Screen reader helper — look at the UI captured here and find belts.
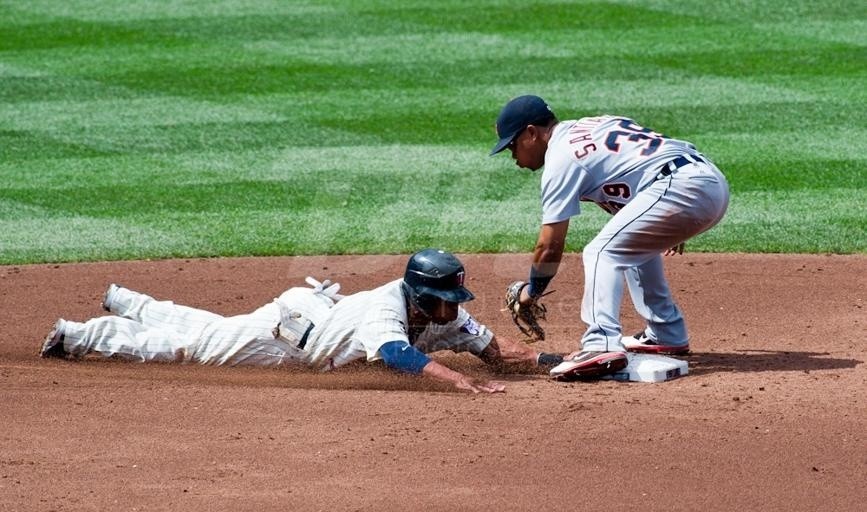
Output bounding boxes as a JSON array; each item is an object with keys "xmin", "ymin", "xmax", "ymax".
[
  {"xmin": 655, "ymin": 154, "xmax": 709, "ymax": 181},
  {"xmin": 295, "ymin": 322, "xmax": 314, "ymax": 351}
]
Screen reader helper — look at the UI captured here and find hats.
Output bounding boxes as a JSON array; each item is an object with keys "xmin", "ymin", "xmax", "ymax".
[{"xmin": 486, "ymin": 96, "xmax": 556, "ymax": 156}]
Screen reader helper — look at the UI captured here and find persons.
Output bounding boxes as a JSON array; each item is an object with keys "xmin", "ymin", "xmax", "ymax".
[
  {"xmin": 488, "ymin": 91, "xmax": 732, "ymax": 381},
  {"xmin": 40, "ymin": 244, "xmax": 571, "ymax": 394}
]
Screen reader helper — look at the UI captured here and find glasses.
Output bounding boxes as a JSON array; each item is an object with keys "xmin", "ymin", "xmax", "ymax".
[{"xmin": 507, "ymin": 130, "xmax": 524, "ymax": 151}]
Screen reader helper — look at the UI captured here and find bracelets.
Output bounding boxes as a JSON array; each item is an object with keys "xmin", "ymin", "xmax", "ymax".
[
  {"xmin": 526, "ymin": 266, "xmax": 557, "ymax": 298},
  {"xmin": 536, "ymin": 350, "xmax": 571, "ymax": 369}
]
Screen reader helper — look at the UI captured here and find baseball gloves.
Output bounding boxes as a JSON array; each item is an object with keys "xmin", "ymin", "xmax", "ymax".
[{"xmin": 505, "ymin": 281, "xmax": 556, "ymax": 344}]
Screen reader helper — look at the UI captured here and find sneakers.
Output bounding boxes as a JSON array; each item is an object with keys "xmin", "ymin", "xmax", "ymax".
[
  {"xmin": 549, "ymin": 347, "xmax": 628, "ymax": 383},
  {"xmin": 39, "ymin": 317, "xmax": 69, "ymax": 358},
  {"xmin": 100, "ymin": 282, "xmax": 121, "ymax": 312},
  {"xmin": 618, "ymin": 330, "xmax": 691, "ymax": 356}
]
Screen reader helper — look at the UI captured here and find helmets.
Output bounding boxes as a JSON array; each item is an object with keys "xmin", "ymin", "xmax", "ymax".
[{"xmin": 402, "ymin": 248, "xmax": 474, "ymax": 305}]
